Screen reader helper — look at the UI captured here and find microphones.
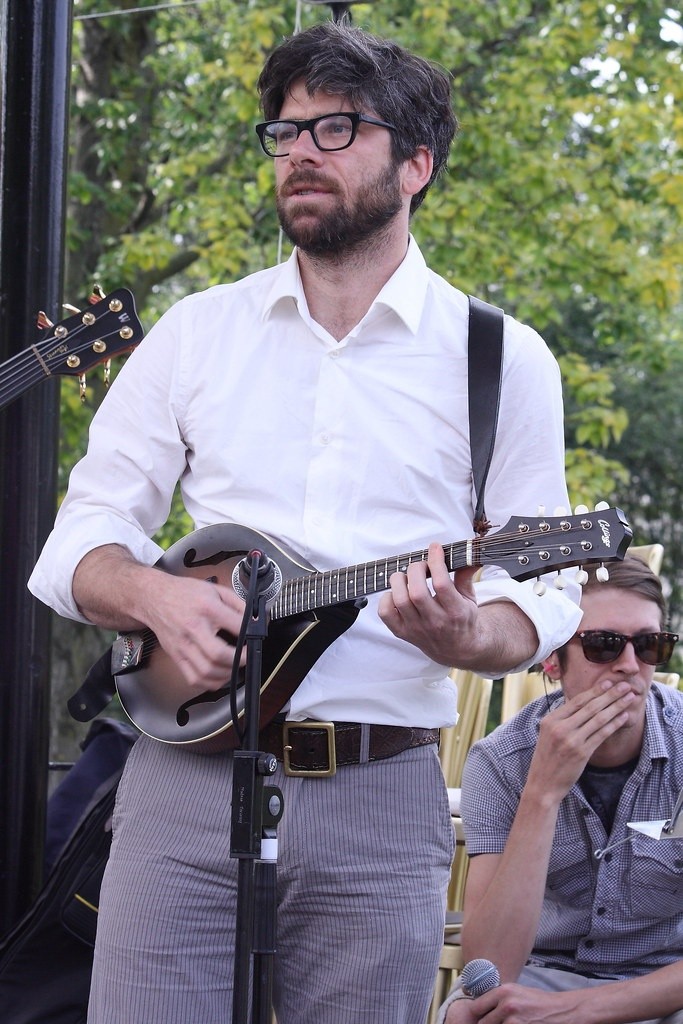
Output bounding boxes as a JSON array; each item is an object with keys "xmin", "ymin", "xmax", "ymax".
[
  {"xmin": 461, "ymin": 958, "xmax": 501, "ymax": 996},
  {"xmin": 232, "ymin": 548, "xmax": 283, "ymax": 604}
]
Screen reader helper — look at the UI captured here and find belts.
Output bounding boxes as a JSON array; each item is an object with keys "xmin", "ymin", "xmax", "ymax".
[{"xmin": 261, "ymin": 725, "xmax": 441, "ymax": 776}]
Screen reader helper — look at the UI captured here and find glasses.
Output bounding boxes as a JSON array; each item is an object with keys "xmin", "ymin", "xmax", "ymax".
[
  {"xmin": 255, "ymin": 110, "xmax": 395, "ymax": 158},
  {"xmin": 575, "ymin": 629, "xmax": 679, "ymax": 666}
]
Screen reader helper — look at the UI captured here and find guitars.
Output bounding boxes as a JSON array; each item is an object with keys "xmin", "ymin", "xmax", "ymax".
[
  {"xmin": 0, "ymin": 283, "xmax": 145, "ymax": 415},
  {"xmin": 114, "ymin": 498, "xmax": 635, "ymax": 758}
]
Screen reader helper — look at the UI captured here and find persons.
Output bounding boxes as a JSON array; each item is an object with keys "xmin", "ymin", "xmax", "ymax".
[
  {"xmin": 26, "ymin": 19, "xmax": 586, "ymax": 1024},
  {"xmin": 434, "ymin": 555, "xmax": 683, "ymax": 1024}
]
